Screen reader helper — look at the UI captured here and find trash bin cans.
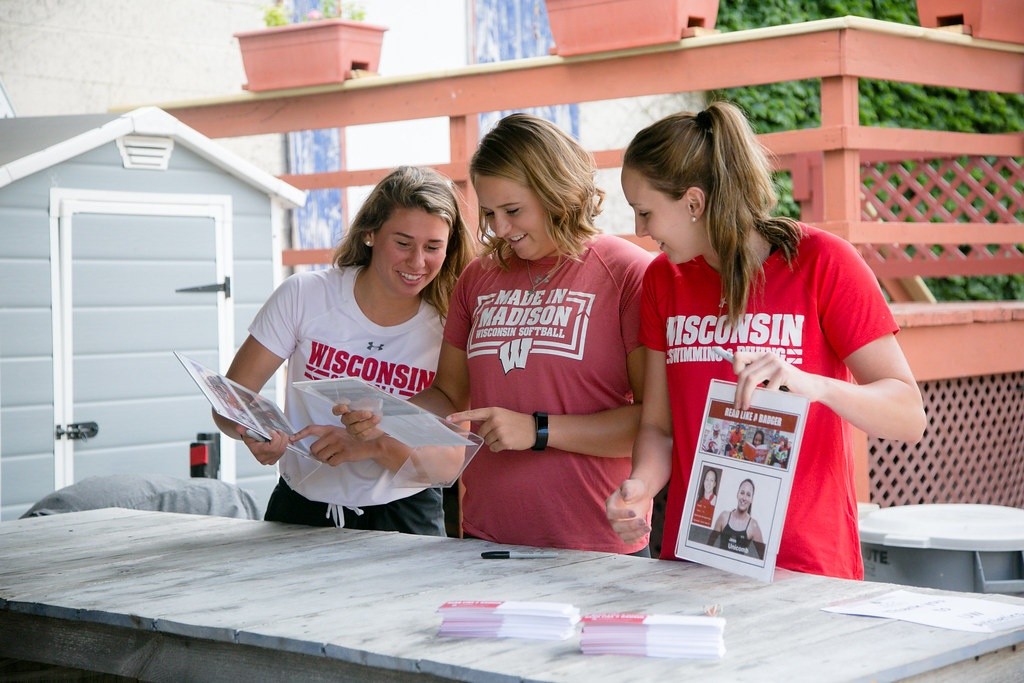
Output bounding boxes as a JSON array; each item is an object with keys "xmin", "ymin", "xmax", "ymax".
[{"xmin": 857, "ymin": 502, "xmax": 1024, "ymax": 598}]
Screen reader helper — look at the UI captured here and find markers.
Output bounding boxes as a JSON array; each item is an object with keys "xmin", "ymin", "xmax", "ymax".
[
  {"xmin": 482, "ymin": 549, "xmax": 560, "ymax": 559},
  {"xmin": 712, "ymin": 345, "xmax": 789, "ymax": 391}
]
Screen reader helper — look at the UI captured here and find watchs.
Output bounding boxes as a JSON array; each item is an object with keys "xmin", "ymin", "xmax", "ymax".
[{"xmin": 533, "ymin": 411, "xmax": 549, "ymax": 452}]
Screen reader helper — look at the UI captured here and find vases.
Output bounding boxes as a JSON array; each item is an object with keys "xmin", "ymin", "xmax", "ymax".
[
  {"xmin": 232, "ymin": 17, "xmax": 388, "ymax": 93},
  {"xmin": 545, "ymin": 0, "xmax": 720, "ymax": 55},
  {"xmin": 913, "ymin": 0, "xmax": 1024, "ymax": 45}
]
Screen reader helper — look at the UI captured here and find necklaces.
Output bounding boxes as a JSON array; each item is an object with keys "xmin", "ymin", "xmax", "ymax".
[{"xmin": 527, "ymin": 238, "xmax": 583, "ymax": 296}]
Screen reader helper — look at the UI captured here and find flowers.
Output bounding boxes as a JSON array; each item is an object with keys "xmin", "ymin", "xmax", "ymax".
[{"xmin": 261, "ymin": 2, "xmax": 366, "ymax": 26}]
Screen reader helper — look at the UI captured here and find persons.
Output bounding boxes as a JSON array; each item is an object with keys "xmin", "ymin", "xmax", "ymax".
[
  {"xmin": 703, "ymin": 422, "xmax": 722, "ymax": 455},
  {"xmin": 211, "ymin": 166, "xmax": 475, "ymax": 537},
  {"xmin": 692, "ymin": 468, "xmax": 717, "ymax": 527},
  {"xmin": 604, "ymin": 101, "xmax": 926, "ymax": 580},
  {"xmin": 770, "ymin": 438, "xmax": 791, "ymax": 469},
  {"xmin": 708, "ymin": 479, "xmax": 766, "ymax": 560},
  {"xmin": 724, "ymin": 424, "xmax": 742, "ymax": 456},
  {"xmin": 741, "ymin": 431, "xmax": 764, "ymax": 460},
  {"xmin": 333, "ymin": 112, "xmax": 656, "ymax": 560}
]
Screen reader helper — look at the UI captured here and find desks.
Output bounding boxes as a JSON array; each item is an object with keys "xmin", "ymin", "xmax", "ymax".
[{"xmin": 0, "ymin": 507, "xmax": 1024, "ymax": 683}]
